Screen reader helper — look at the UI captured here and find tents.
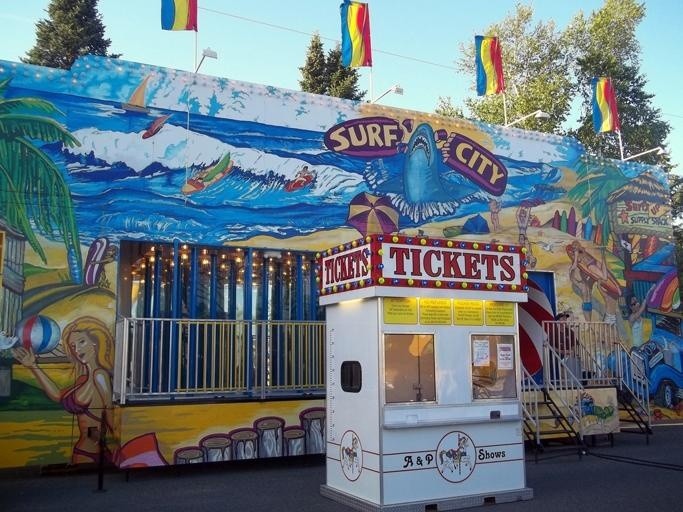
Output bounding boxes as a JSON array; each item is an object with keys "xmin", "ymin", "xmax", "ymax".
[{"xmin": 459, "ymin": 214, "xmax": 489, "ymax": 234}]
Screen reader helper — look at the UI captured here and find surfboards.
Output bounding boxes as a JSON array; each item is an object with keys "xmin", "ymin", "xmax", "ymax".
[
  {"xmin": 142, "ymin": 116, "xmax": 168, "ymax": 138},
  {"xmin": 566, "ymin": 244, "xmax": 622, "ymax": 297},
  {"xmin": 285, "ymin": 175, "xmax": 313, "ymax": 192},
  {"xmin": 182, "ymin": 160, "xmax": 234, "ymax": 192}
]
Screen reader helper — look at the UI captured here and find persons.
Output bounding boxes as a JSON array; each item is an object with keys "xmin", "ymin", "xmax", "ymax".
[
  {"xmin": 290, "ymin": 165, "xmax": 309, "ymax": 188},
  {"xmin": 488, "ymin": 199, "xmax": 501, "ymax": 233},
  {"xmin": 514, "ymin": 203, "xmax": 529, "ymax": 237},
  {"xmin": 6, "ymin": 315, "xmax": 116, "ymax": 465},
  {"xmin": 594, "ymin": 279, "xmax": 620, "ymax": 334},
  {"xmin": 415, "ymin": 229, "xmax": 429, "ymax": 238},
  {"xmin": 625, "ymin": 282, "xmax": 658, "ymax": 351},
  {"xmin": 568, "ymin": 239, "xmax": 624, "ymax": 298},
  {"xmin": 88, "ymin": 244, "xmax": 118, "ymax": 288},
  {"xmin": 522, "ymin": 239, "xmax": 538, "ymax": 268},
  {"xmin": 517, "ymin": 233, "xmax": 527, "ymax": 254},
  {"xmin": 568, "ymin": 267, "xmax": 600, "ymax": 322}
]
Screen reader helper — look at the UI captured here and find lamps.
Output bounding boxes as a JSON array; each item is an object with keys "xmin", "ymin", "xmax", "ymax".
[{"xmin": 194, "ymin": 47, "xmax": 217, "ymax": 74}]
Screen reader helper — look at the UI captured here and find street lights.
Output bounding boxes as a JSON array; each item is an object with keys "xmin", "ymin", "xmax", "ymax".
[{"xmin": 195, "ymin": 48, "xmax": 218, "ymax": 71}]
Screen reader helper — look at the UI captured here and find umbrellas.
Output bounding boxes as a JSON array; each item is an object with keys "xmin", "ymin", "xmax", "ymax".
[{"xmin": 344, "ymin": 191, "xmax": 401, "ymax": 238}]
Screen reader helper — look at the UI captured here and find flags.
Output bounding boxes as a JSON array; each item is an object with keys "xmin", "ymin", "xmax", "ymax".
[
  {"xmin": 158, "ymin": 0, "xmax": 200, "ymax": 33},
  {"xmin": 473, "ymin": 35, "xmax": 504, "ymax": 97},
  {"xmin": 335, "ymin": 0, "xmax": 373, "ymax": 69},
  {"xmin": 586, "ymin": 75, "xmax": 621, "ymax": 133}
]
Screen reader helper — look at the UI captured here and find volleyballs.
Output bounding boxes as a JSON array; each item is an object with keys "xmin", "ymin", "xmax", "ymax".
[{"xmin": 18, "ymin": 314, "xmax": 60, "ymax": 355}]
